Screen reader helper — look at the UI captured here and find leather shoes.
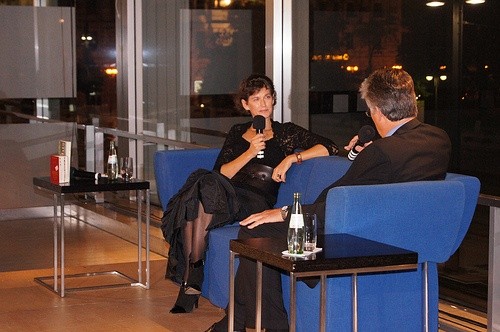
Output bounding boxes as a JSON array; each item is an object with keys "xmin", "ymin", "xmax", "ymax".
[{"xmin": 205, "ymin": 322, "xmax": 246, "ymax": 332}]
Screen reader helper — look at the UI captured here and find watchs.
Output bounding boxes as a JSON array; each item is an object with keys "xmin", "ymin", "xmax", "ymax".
[{"xmin": 281, "ymin": 205, "xmax": 289, "ymax": 220}]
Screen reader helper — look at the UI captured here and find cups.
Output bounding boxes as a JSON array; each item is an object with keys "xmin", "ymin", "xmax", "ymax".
[
  {"xmin": 120, "ymin": 157, "xmax": 133, "ymax": 182},
  {"xmin": 303, "ymin": 212, "xmax": 317, "ymax": 251}
]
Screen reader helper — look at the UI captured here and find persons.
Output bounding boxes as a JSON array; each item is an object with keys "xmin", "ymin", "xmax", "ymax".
[
  {"xmin": 161, "ymin": 74, "xmax": 338, "ymax": 313},
  {"xmin": 205, "ymin": 67, "xmax": 451, "ymax": 332}
]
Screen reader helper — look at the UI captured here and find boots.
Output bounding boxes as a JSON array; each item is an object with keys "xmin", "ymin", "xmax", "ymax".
[
  {"xmin": 183, "ymin": 253, "xmax": 206, "ymax": 295},
  {"xmin": 170, "ymin": 280, "xmax": 200, "ymax": 314}
]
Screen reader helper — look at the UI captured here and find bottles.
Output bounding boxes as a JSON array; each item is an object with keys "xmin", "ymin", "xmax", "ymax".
[
  {"xmin": 107, "ymin": 140, "xmax": 118, "ymax": 180},
  {"xmin": 287, "ymin": 192, "xmax": 305, "ymax": 255}
]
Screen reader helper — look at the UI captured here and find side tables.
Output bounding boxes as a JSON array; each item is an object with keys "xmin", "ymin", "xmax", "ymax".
[
  {"xmin": 32, "ymin": 174, "xmax": 151, "ymax": 298},
  {"xmin": 228, "ymin": 233, "xmax": 419, "ymax": 332}
]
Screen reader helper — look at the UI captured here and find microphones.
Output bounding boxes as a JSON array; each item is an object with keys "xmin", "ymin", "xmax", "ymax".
[
  {"xmin": 253, "ymin": 115, "xmax": 266, "ymax": 159},
  {"xmin": 69, "ymin": 167, "xmax": 101, "ymax": 179},
  {"xmin": 348, "ymin": 125, "xmax": 375, "ymax": 161}
]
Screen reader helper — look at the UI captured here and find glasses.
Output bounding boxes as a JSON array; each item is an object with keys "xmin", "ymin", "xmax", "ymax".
[{"xmin": 365, "ymin": 106, "xmax": 372, "ymax": 117}]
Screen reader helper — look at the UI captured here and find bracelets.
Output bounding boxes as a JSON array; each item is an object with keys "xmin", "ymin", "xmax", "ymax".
[{"xmin": 296, "ymin": 152, "xmax": 301, "ymax": 162}]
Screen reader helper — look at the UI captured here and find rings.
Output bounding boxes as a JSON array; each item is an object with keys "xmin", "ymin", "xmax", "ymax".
[{"xmin": 277, "ymin": 174, "xmax": 281, "ymax": 179}]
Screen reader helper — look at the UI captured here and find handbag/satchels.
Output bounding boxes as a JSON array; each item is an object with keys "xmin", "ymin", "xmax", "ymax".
[{"xmin": 237, "ymin": 163, "xmax": 273, "ymax": 189}]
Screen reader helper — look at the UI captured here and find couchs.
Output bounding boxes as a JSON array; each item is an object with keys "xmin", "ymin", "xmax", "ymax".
[{"xmin": 152, "ymin": 148, "xmax": 481, "ymax": 332}]
